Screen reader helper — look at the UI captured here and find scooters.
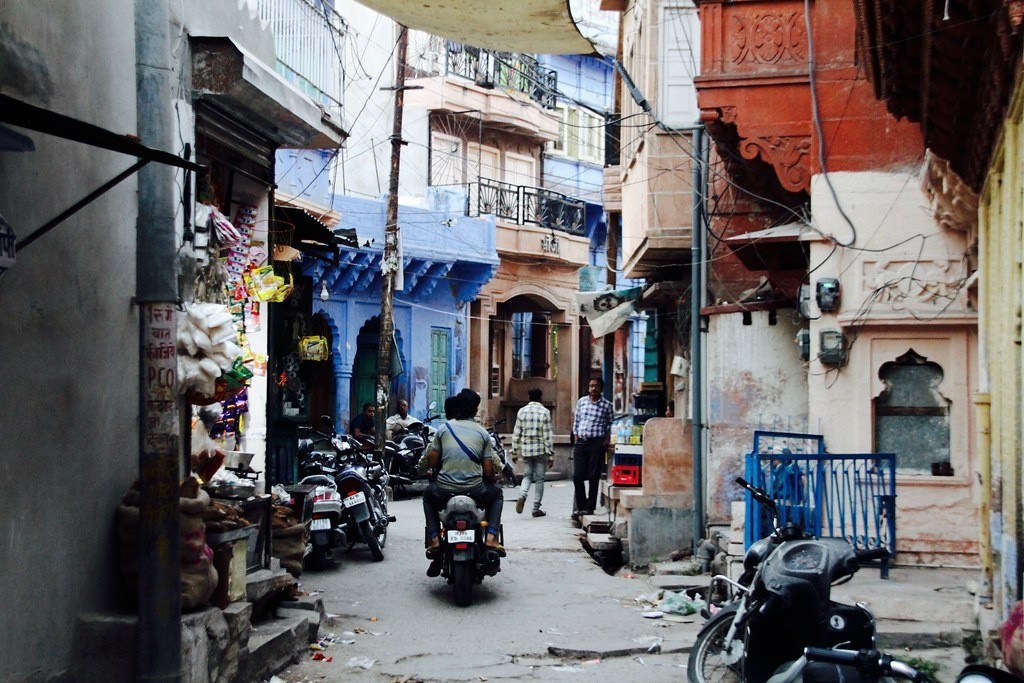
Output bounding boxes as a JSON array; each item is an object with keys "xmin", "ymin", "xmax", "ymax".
[
  {"xmin": 685, "ymin": 476, "xmax": 1024, "ymax": 683},
  {"xmin": 485, "ymin": 417, "xmax": 520, "ymax": 487}
]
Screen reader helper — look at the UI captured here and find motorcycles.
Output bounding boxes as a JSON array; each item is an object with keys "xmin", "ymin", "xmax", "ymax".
[
  {"xmin": 296, "ymin": 414, "xmax": 397, "ymax": 568},
  {"xmin": 427, "ymin": 489, "xmax": 506, "ymax": 607},
  {"xmin": 357, "ymin": 401, "xmax": 442, "ymax": 497}
]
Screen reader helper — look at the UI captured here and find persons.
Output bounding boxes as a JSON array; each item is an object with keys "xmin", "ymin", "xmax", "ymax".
[
  {"xmin": 385, "ymin": 399, "xmax": 437, "ymax": 440},
  {"xmin": 350, "ymin": 403, "xmax": 375, "ymax": 443},
  {"xmin": 1000, "ymin": 599, "xmax": 1024, "ymax": 677},
  {"xmin": 512, "ymin": 388, "xmax": 554, "ymax": 517},
  {"xmin": 771, "ymin": 445, "xmax": 806, "ymax": 501},
  {"xmin": 573, "ymin": 376, "xmax": 613, "ymax": 513},
  {"xmin": 416, "ymin": 389, "xmax": 506, "ymax": 577},
  {"xmin": 665, "ymin": 400, "xmax": 674, "ymax": 418}
]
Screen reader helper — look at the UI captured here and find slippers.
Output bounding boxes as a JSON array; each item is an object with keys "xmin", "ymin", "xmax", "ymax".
[
  {"xmin": 426, "ymin": 544, "xmax": 440, "ymax": 559},
  {"xmin": 490, "ymin": 543, "xmax": 506, "ymax": 557}
]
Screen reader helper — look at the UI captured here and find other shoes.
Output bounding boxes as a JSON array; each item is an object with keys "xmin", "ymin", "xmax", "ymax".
[
  {"xmin": 516, "ymin": 496, "xmax": 525, "ymax": 514},
  {"xmin": 533, "ymin": 510, "xmax": 545, "ymax": 517},
  {"xmin": 427, "ymin": 559, "xmax": 443, "ymax": 577}
]
